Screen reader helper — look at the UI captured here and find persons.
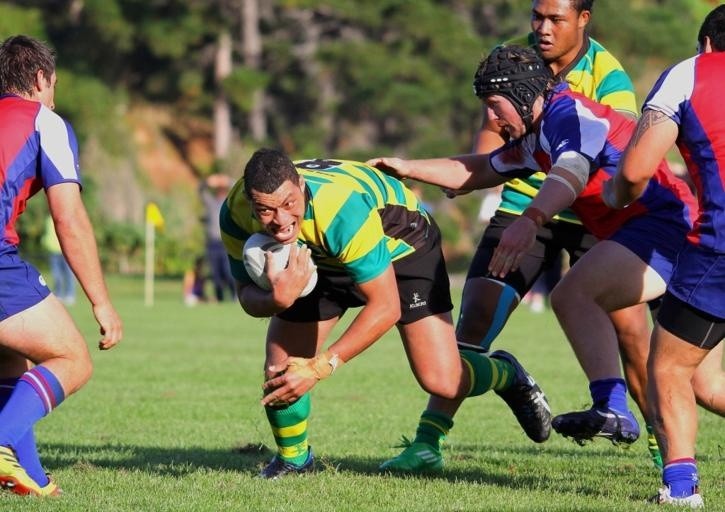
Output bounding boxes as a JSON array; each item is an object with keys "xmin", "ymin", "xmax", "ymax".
[
  {"xmin": 375, "ymin": 0, "xmax": 673, "ymax": 476},
  {"xmin": 219, "ymin": 147, "xmax": 556, "ymax": 483},
  {"xmin": 365, "ymin": 40, "xmax": 724, "ymax": 446},
  {"xmin": 201, "ymin": 171, "xmax": 234, "ymax": 307},
  {"xmin": 602, "ymin": 0, "xmax": 725, "ymax": 511},
  {"xmin": 182, "ymin": 256, "xmax": 209, "ymax": 305},
  {"xmin": 1, "ymin": 34, "xmax": 123, "ymax": 498}
]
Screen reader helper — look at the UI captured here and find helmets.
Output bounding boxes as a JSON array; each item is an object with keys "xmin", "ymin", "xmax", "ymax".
[{"xmin": 472, "ymin": 43, "xmax": 552, "ymax": 122}]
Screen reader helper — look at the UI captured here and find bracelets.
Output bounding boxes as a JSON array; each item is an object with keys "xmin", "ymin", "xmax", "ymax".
[
  {"xmin": 521, "ymin": 206, "xmax": 548, "ymax": 229},
  {"xmin": 610, "ymin": 193, "xmax": 629, "ymax": 209}
]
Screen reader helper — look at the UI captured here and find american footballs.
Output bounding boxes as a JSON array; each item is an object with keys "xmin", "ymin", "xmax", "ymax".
[{"xmin": 242, "ymin": 231, "xmax": 319, "ymax": 299}]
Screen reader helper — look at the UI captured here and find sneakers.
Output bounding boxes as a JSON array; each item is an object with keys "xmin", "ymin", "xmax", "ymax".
[
  {"xmin": 256, "ymin": 448, "xmax": 316, "ymax": 481},
  {"xmin": 651, "ymin": 489, "xmax": 704, "ymax": 507},
  {"xmin": 378, "ymin": 434, "xmax": 445, "ymax": 476},
  {"xmin": 490, "ymin": 348, "xmax": 551, "ymax": 442},
  {"xmin": 551, "ymin": 402, "xmax": 638, "ymax": 448},
  {"xmin": 647, "ymin": 425, "xmax": 664, "ymax": 471},
  {"xmin": 0, "ymin": 445, "xmax": 66, "ymax": 497}
]
